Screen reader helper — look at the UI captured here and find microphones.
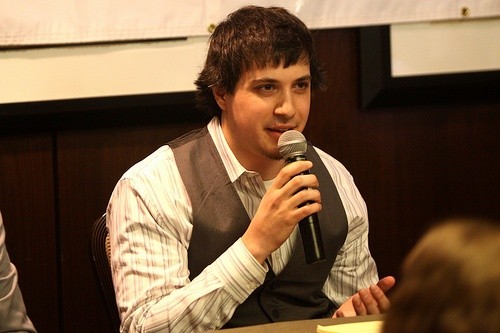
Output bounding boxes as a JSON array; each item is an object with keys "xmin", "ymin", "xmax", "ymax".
[{"xmin": 278, "ymin": 130, "xmax": 327, "ymax": 264}]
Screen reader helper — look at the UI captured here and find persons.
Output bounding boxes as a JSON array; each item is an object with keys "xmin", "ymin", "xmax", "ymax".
[{"xmin": 105, "ymin": 6, "xmax": 395, "ymax": 333}]
